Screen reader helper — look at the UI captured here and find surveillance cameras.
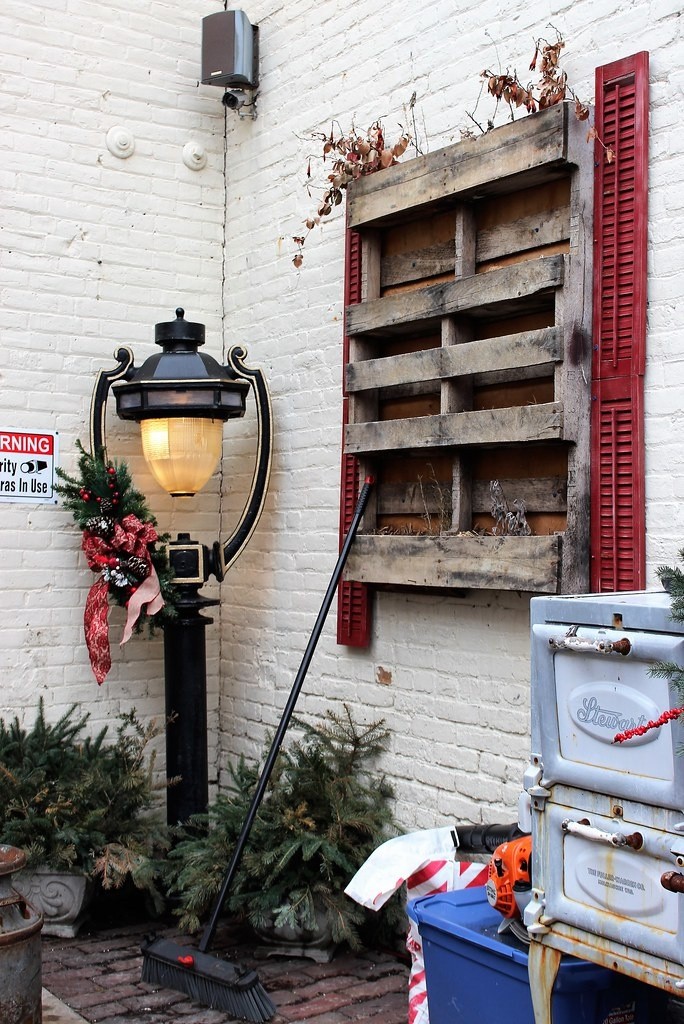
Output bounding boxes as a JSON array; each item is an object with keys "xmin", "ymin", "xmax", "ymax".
[{"xmin": 222, "ymin": 89, "xmax": 248, "ymax": 110}]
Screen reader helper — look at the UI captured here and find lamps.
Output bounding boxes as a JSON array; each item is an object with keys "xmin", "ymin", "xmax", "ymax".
[{"xmin": 111, "ymin": 307, "xmax": 250, "ymax": 497}]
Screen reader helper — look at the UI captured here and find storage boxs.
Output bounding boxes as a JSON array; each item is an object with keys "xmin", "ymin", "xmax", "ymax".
[{"xmin": 405, "ymin": 887, "xmax": 642, "ymax": 1024}]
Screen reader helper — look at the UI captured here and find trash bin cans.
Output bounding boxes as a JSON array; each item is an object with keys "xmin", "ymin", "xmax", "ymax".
[{"xmin": 406, "ymin": 885, "xmax": 637, "ymax": 1024}]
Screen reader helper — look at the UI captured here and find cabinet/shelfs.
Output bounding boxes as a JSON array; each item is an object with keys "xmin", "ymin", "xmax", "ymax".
[{"xmin": 512, "ymin": 586, "xmax": 684, "ymax": 1023}]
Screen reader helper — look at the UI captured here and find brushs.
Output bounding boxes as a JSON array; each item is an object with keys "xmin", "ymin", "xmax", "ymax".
[{"xmin": 139, "ymin": 476, "xmax": 372, "ymax": 1023}]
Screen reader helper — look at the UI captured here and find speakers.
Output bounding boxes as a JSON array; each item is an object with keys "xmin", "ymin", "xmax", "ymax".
[{"xmin": 202, "ymin": 10, "xmax": 253, "ymax": 86}]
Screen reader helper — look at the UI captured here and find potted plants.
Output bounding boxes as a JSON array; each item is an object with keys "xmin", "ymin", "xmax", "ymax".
[
  {"xmin": 0, "ymin": 693, "xmax": 182, "ymax": 940},
  {"xmin": 135, "ymin": 700, "xmax": 413, "ymax": 964}
]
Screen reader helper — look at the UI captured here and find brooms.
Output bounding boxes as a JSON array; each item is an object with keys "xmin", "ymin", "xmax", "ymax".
[{"xmin": 142, "ymin": 478, "xmax": 375, "ymax": 1022}]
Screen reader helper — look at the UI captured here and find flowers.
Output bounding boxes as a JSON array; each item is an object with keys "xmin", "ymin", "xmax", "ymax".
[{"xmin": 49, "ymin": 437, "xmax": 181, "ymax": 684}]
